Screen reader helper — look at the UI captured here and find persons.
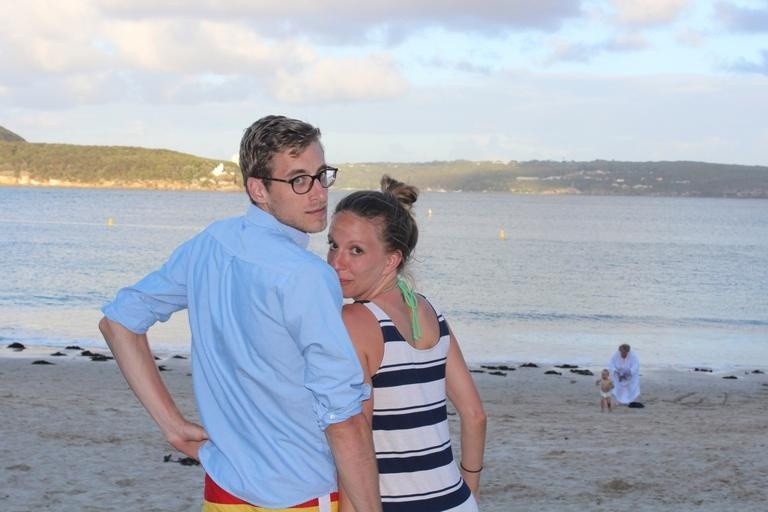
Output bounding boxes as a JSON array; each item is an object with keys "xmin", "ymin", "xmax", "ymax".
[
  {"xmin": 596, "ymin": 370, "xmax": 616, "ymax": 412},
  {"xmin": 606, "ymin": 342, "xmax": 642, "ymax": 407},
  {"xmin": 98, "ymin": 115, "xmax": 384, "ymax": 511},
  {"xmin": 326, "ymin": 171, "xmax": 489, "ymax": 512}
]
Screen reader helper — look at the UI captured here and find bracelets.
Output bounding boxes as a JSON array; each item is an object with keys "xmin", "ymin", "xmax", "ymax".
[{"xmin": 460, "ymin": 461, "xmax": 484, "ymax": 474}]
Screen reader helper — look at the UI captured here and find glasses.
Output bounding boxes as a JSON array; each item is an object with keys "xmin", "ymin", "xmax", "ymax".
[{"xmin": 245, "ymin": 165, "xmax": 339, "ymax": 198}]
